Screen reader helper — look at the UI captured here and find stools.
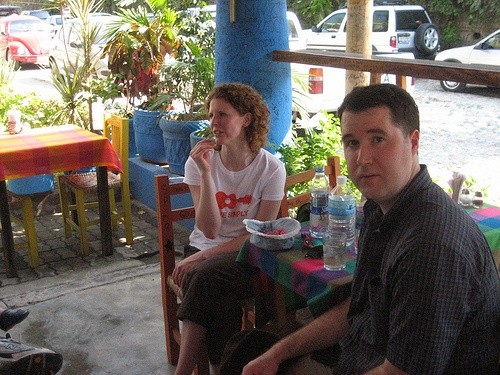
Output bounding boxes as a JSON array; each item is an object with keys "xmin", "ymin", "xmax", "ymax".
[{"xmin": 6, "ymin": 174, "xmax": 57, "ymax": 270}]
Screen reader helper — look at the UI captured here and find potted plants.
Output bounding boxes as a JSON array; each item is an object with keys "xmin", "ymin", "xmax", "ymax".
[{"xmin": 104, "ymin": 4, "xmax": 214, "ymax": 175}]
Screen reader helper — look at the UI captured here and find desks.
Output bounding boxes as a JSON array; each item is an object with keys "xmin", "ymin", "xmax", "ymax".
[
  {"xmin": 246, "ymin": 198, "xmax": 500, "ymax": 336},
  {"xmin": 0, "ymin": 124, "xmax": 120, "ymax": 264}
]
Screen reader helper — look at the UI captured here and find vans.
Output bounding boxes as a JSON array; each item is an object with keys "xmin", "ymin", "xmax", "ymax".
[
  {"xmin": 21, "ymin": 10, "xmax": 49, "ymax": 20},
  {"xmin": 0, "ymin": 6, "xmax": 21, "ymax": 16}
]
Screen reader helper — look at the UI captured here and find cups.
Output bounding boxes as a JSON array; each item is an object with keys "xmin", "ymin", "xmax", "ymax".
[{"xmin": 322, "ymin": 231, "xmax": 347, "ymax": 273}]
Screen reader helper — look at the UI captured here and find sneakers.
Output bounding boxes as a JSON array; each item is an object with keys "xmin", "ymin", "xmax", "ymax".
[{"xmin": 0, "ymin": 332, "xmax": 63, "ymax": 375}]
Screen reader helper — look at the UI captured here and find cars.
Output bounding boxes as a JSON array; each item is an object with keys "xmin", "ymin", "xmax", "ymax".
[
  {"xmin": 50, "ymin": 12, "xmax": 176, "ymax": 91},
  {"xmin": 0, "ymin": 16, "xmax": 57, "ymax": 71},
  {"xmin": 49, "ymin": 15, "xmax": 63, "ymax": 39},
  {"xmin": 435, "ymin": 29, "xmax": 500, "ymax": 92}
]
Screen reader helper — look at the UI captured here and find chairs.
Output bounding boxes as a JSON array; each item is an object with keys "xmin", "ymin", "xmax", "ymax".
[
  {"xmin": 60, "ymin": 116, "xmax": 133, "ymax": 254},
  {"xmin": 155, "ymin": 171, "xmax": 255, "ymax": 375},
  {"xmin": 272, "ymin": 155, "xmax": 341, "ymax": 222}
]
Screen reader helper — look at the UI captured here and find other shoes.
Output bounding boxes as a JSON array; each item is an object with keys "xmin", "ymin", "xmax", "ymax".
[{"xmin": 0, "ymin": 308, "xmax": 30, "ymax": 332}]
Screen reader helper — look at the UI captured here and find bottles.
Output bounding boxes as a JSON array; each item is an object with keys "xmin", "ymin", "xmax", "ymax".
[
  {"xmin": 310, "ymin": 166, "xmax": 331, "ymax": 238},
  {"xmin": 328, "ymin": 176, "xmax": 354, "ymax": 245},
  {"xmin": 353, "ymin": 198, "xmax": 367, "ymax": 255}
]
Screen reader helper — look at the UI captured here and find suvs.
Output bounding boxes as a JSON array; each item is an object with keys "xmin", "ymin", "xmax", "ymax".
[{"xmin": 303, "ymin": 4, "xmax": 440, "ymax": 62}]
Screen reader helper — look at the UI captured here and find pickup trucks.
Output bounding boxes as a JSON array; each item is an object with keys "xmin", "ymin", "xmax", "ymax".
[{"xmin": 201, "ymin": 5, "xmax": 416, "ymax": 115}]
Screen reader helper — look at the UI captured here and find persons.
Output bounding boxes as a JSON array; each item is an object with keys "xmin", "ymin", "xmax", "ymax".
[
  {"xmin": 242, "ymin": 84, "xmax": 500, "ymax": 375},
  {"xmin": 171, "ymin": 82, "xmax": 287, "ymax": 375},
  {"xmin": 0, "ymin": 306, "xmax": 63, "ymax": 375}
]
